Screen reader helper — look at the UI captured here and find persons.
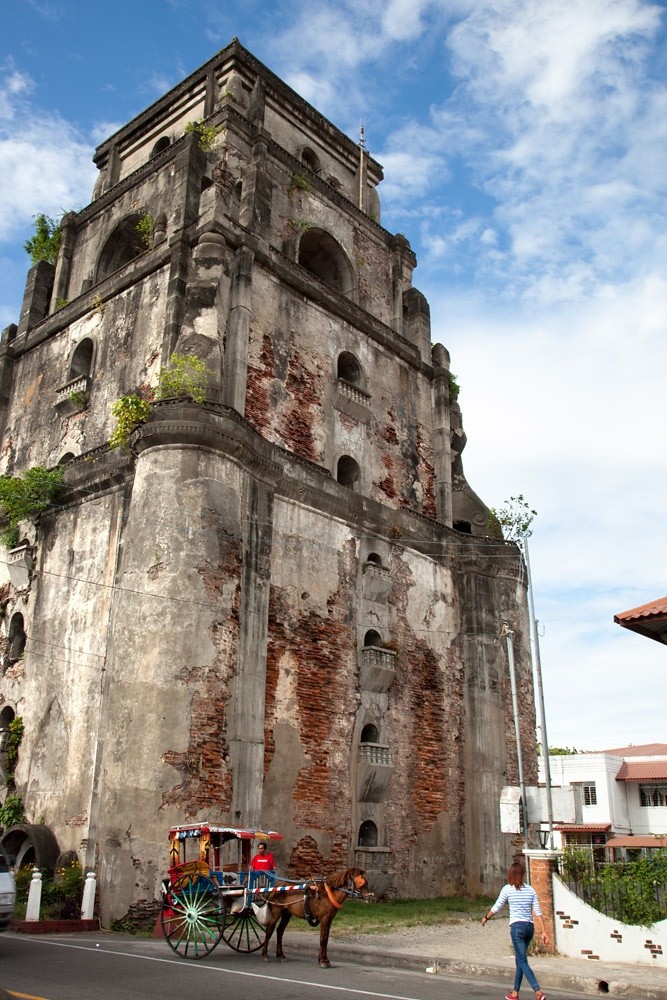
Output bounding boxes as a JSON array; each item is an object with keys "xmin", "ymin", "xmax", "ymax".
[
  {"xmin": 249, "ymin": 842, "xmax": 276, "ymax": 872},
  {"xmin": 481, "ymin": 863, "xmax": 550, "ymax": 1000}
]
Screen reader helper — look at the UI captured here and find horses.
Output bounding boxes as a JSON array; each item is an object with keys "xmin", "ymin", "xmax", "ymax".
[{"xmin": 259, "ymin": 860, "xmax": 376, "ymax": 969}]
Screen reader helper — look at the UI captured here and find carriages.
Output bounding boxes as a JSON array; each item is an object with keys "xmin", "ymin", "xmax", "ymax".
[{"xmin": 158, "ymin": 821, "xmax": 378, "ymax": 968}]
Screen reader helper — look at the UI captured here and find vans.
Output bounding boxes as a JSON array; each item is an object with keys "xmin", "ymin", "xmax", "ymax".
[{"xmin": 0, "ymin": 846, "xmax": 17, "ymax": 927}]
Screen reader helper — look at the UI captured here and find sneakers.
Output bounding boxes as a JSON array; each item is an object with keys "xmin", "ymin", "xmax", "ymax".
[
  {"xmin": 536, "ymin": 992, "xmax": 547, "ymax": 1000},
  {"xmin": 504, "ymin": 992, "xmax": 519, "ymax": 1000}
]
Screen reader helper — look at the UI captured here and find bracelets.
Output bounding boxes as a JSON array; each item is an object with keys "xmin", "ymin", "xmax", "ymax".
[{"xmin": 485, "ymin": 914, "xmax": 489, "ymax": 920}]
